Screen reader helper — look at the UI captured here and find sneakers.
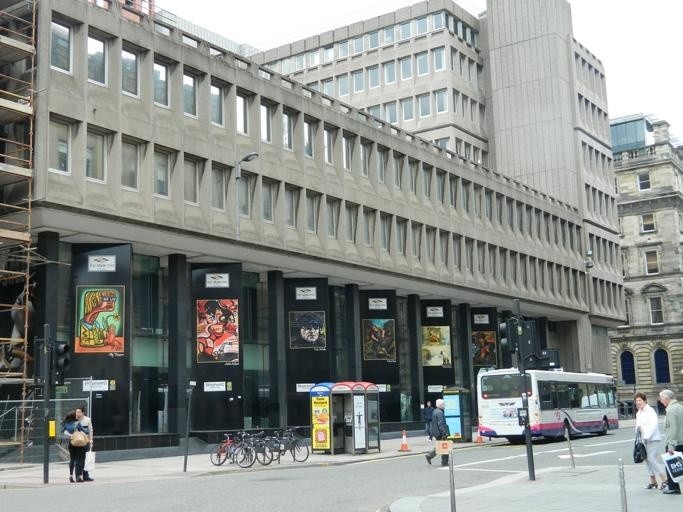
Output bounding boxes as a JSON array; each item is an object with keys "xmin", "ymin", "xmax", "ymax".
[
  {"xmin": 84, "ymin": 477, "xmax": 93, "ymax": 481},
  {"xmin": 663, "ymin": 489, "xmax": 680, "ymax": 494},
  {"xmin": 77, "ymin": 479, "xmax": 84, "ymax": 482},
  {"xmin": 426, "ymin": 454, "xmax": 431, "ymax": 464},
  {"xmin": 69, "ymin": 477, "xmax": 75, "ymax": 482}
]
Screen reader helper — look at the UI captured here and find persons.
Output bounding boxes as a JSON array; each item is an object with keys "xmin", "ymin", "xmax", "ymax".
[
  {"xmin": 425, "ymin": 398, "xmax": 451, "ymax": 467},
  {"xmin": 659, "ymin": 389, "xmax": 683, "ymax": 494},
  {"xmin": 291, "ymin": 314, "xmax": 323, "ymax": 346},
  {"xmin": 423, "ymin": 400, "xmax": 434, "ymax": 436},
  {"xmin": 61, "ymin": 410, "xmax": 88, "ymax": 483},
  {"xmin": 76, "ymin": 405, "xmax": 93, "ymax": 481},
  {"xmin": 634, "ymin": 392, "xmax": 670, "ymax": 490}
]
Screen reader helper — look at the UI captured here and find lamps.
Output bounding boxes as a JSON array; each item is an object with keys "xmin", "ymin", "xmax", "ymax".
[{"xmin": 235, "ymin": 152, "xmax": 258, "ymax": 179}]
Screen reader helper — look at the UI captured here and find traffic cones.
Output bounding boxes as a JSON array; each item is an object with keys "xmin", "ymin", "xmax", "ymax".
[{"xmin": 396, "ymin": 429, "xmax": 410, "ymax": 453}]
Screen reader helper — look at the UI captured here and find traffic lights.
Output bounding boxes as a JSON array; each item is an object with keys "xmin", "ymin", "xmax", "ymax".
[
  {"xmin": 57, "ymin": 343, "xmax": 69, "ymax": 368},
  {"xmin": 499, "ymin": 318, "xmax": 514, "ymax": 352}
]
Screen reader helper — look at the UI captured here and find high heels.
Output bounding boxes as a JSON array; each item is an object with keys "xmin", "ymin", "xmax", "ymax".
[
  {"xmin": 645, "ymin": 482, "xmax": 658, "ymax": 489},
  {"xmin": 660, "ymin": 480, "xmax": 667, "ymax": 490}
]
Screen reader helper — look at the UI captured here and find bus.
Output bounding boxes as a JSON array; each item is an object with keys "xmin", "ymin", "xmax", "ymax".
[{"xmin": 475, "ymin": 366, "xmax": 620, "ymax": 442}]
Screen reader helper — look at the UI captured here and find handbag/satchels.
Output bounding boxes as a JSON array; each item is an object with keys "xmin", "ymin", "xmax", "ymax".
[
  {"xmin": 633, "ymin": 444, "xmax": 646, "ymax": 462},
  {"xmin": 69, "ymin": 432, "xmax": 88, "ymax": 447},
  {"xmin": 432, "ymin": 425, "xmax": 450, "ymax": 438},
  {"xmin": 83, "ymin": 452, "xmax": 96, "ymax": 471},
  {"xmin": 661, "ymin": 451, "xmax": 683, "ymax": 483},
  {"xmin": 436, "ymin": 440, "xmax": 453, "ymax": 454}
]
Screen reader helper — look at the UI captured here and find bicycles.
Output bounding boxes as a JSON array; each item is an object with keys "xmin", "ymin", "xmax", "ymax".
[{"xmin": 207, "ymin": 426, "xmax": 310, "ymax": 469}]
